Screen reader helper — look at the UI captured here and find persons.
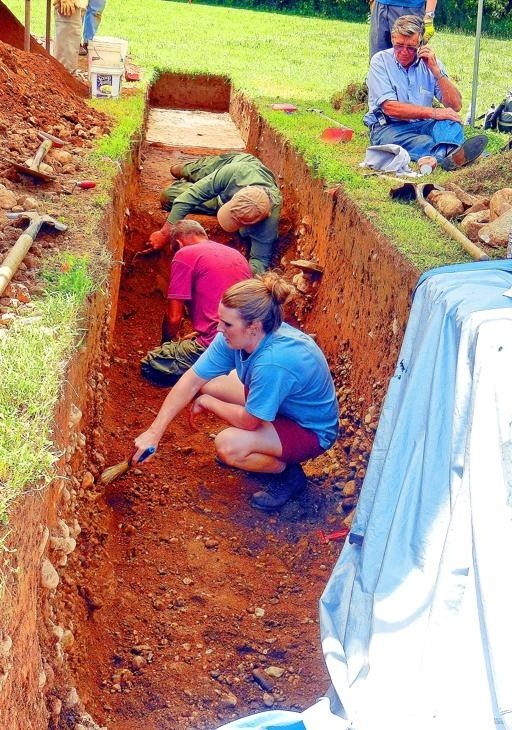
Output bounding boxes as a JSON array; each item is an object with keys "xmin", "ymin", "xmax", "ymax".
[
  {"xmin": 125, "ymin": 269, "xmax": 343, "ymax": 513},
  {"xmin": 136, "ymin": 218, "xmax": 258, "ymax": 388},
  {"xmin": 48, "ymin": 0, "xmax": 90, "ymax": 76},
  {"xmin": 73, "ymin": 0, "xmax": 108, "ymax": 55},
  {"xmin": 142, "ymin": 150, "xmax": 286, "ymax": 278},
  {"xmin": 365, "ymin": 0, "xmax": 438, "ymax": 72},
  {"xmin": 361, "ymin": 14, "xmax": 490, "ymax": 173}
]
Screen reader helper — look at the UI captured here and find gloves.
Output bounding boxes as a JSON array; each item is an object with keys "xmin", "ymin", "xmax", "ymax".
[
  {"xmin": 422, "ymin": 17, "xmax": 435, "ymax": 45},
  {"xmin": 57, "ymin": 0, "xmax": 79, "ymax": 18},
  {"xmin": 141, "ymin": 221, "xmax": 175, "ymax": 256}
]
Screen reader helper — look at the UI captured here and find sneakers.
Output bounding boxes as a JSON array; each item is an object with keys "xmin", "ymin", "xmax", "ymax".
[
  {"xmin": 248, "ymin": 465, "xmax": 307, "ymax": 512},
  {"xmin": 440, "ymin": 134, "xmax": 488, "ymax": 171}
]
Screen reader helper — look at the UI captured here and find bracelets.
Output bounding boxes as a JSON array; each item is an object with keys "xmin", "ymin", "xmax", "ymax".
[
  {"xmin": 433, "ymin": 70, "xmax": 448, "ymax": 82},
  {"xmin": 433, "ymin": 107, "xmax": 438, "ymax": 120}
]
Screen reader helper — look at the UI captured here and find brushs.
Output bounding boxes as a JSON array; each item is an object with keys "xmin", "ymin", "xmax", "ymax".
[{"xmin": 102, "ymin": 448, "xmax": 155, "ymax": 486}]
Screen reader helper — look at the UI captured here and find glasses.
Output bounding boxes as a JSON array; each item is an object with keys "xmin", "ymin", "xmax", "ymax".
[{"xmin": 391, "ymin": 39, "xmax": 422, "ymax": 52}]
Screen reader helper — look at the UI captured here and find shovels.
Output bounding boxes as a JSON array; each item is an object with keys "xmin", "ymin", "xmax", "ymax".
[
  {"xmin": 390, "ymin": 183, "xmax": 489, "ymax": 261},
  {"xmin": 3, "ymin": 131, "xmax": 63, "ymax": 183},
  {"xmin": 0, "ymin": 211, "xmax": 67, "ymax": 296}
]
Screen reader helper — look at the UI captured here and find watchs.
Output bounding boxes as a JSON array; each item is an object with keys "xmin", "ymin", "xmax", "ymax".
[{"xmin": 425, "ymin": 11, "xmax": 435, "ymax": 18}]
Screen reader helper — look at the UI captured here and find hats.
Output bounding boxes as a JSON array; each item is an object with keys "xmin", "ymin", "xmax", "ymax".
[{"xmin": 215, "ymin": 184, "xmax": 270, "ymax": 234}]
